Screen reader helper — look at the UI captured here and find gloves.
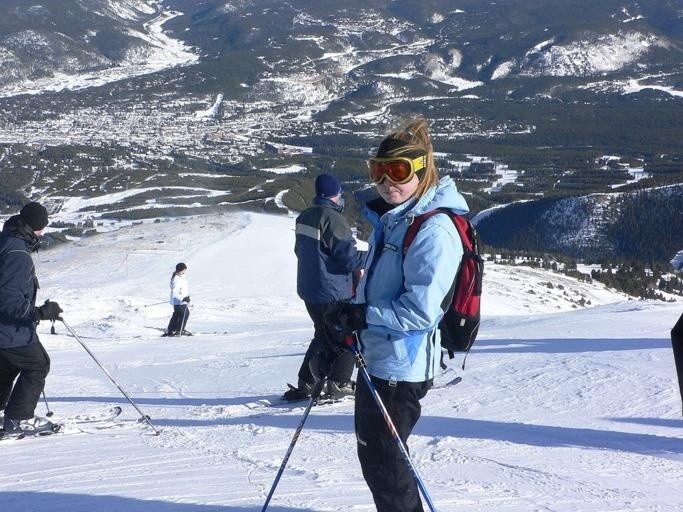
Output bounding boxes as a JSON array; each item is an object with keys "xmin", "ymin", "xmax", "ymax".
[
  {"xmin": 324, "ymin": 300, "xmax": 368, "ymax": 351},
  {"xmin": 34, "ymin": 302, "xmax": 64, "ymax": 323},
  {"xmin": 182, "ymin": 297, "xmax": 190, "ymax": 303}
]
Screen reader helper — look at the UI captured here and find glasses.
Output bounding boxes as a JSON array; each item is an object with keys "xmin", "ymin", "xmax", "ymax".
[{"xmin": 368, "ymin": 154, "xmax": 427, "ymax": 185}]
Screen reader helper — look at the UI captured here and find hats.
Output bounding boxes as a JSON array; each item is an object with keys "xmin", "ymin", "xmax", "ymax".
[
  {"xmin": 176, "ymin": 263, "xmax": 187, "ymax": 272},
  {"xmin": 315, "ymin": 174, "xmax": 341, "ymax": 197},
  {"xmin": 20, "ymin": 202, "xmax": 48, "ymax": 232}
]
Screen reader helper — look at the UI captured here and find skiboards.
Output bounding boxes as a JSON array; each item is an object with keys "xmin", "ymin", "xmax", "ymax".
[
  {"xmin": 276, "ymin": 366, "xmax": 462, "ymax": 407},
  {"xmin": 144, "ymin": 326, "xmax": 231, "ymax": 336},
  {"xmin": 0, "ymin": 404, "xmax": 150, "ymax": 440}
]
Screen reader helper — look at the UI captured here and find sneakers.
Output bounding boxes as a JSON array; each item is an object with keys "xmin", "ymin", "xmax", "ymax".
[
  {"xmin": 3, "ymin": 415, "xmax": 54, "ymax": 434},
  {"xmin": 298, "ymin": 377, "xmax": 316, "ymax": 396},
  {"xmin": 317, "ymin": 382, "xmax": 355, "ymax": 400}
]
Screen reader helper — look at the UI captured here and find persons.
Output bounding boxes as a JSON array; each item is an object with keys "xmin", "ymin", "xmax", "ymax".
[
  {"xmin": 323, "ymin": 115, "xmax": 471, "ymax": 511},
  {"xmin": 168, "ymin": 263, "xmax": 190, "ymax": 335},
  {"xmin": 0, "ymin": 202, "xmax": 63, "ymax": 436},
  {"xmin": 294, "ymin": 173, "xmax": 364, "ymax": 399}
]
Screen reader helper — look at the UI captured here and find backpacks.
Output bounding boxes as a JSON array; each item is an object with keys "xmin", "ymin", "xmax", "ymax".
[{"xmin": 401, "ymin": 205, "xmax": 484, "ymax": 352}]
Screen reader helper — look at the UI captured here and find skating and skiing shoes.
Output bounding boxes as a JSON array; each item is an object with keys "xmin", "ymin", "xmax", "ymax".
[{"xmin": 168, "ymin": 329, "xmax": 191, "ymax": 335}]
